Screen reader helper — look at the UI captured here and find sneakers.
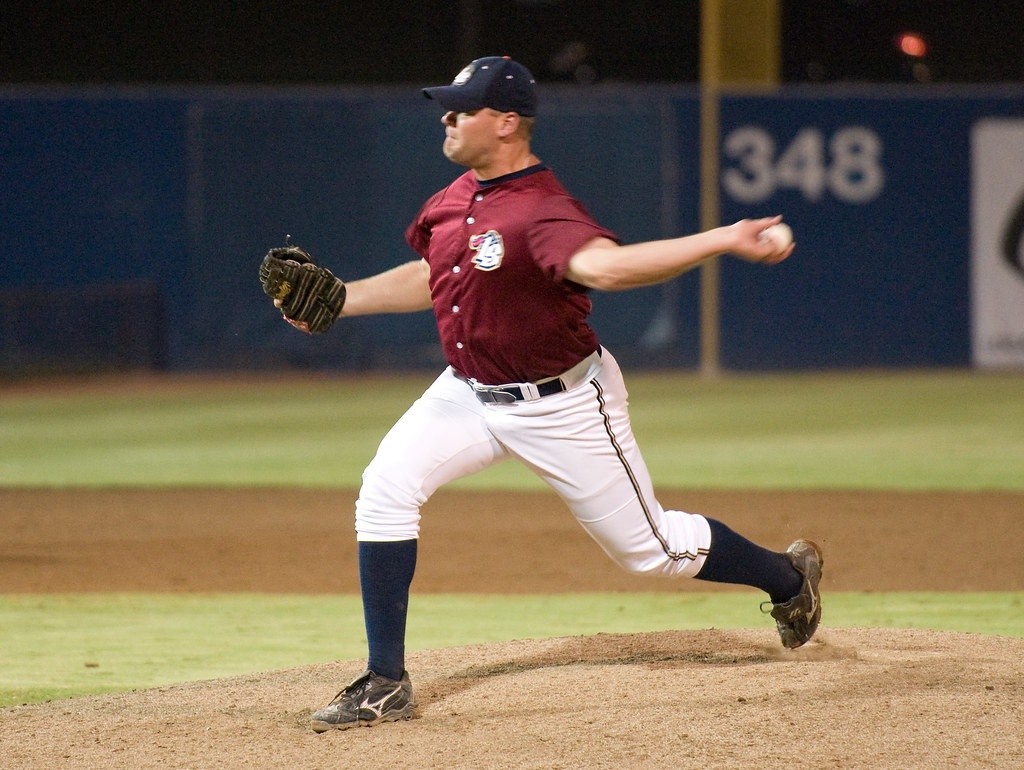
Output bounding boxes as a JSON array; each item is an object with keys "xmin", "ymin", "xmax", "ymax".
[
  {"xmin": 310, "ymin": 669, "xmax": 420, "ymax": 735},
  {"xmin": 760, "ymin": 539, "xmax": 824, "ymax": 649}
]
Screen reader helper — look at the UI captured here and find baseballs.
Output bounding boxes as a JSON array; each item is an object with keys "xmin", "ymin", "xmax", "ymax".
[{"xmin": 756, "ymin": 222, "xmax": 794, "ymax": 255}]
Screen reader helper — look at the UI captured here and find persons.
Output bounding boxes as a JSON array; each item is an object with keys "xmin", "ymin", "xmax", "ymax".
[{"xmin": 274, "ymin": 58, "xmax": 822, "ymax": 730}]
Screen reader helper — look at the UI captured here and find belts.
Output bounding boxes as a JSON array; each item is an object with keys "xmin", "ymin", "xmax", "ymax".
[{"xmin": 453, "ymin": 346, "xmax": 602, "ymax": 405}]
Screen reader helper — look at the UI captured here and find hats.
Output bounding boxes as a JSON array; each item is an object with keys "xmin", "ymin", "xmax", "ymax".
[{"xmin": 421, "ymin": 56, "xmax": 537, "ymax": 117}]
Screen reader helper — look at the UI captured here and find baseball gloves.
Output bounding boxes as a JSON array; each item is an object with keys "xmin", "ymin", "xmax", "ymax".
[{"xmin": 259, "ymin": 246, "xmax": 347, "ymax": 337}]
